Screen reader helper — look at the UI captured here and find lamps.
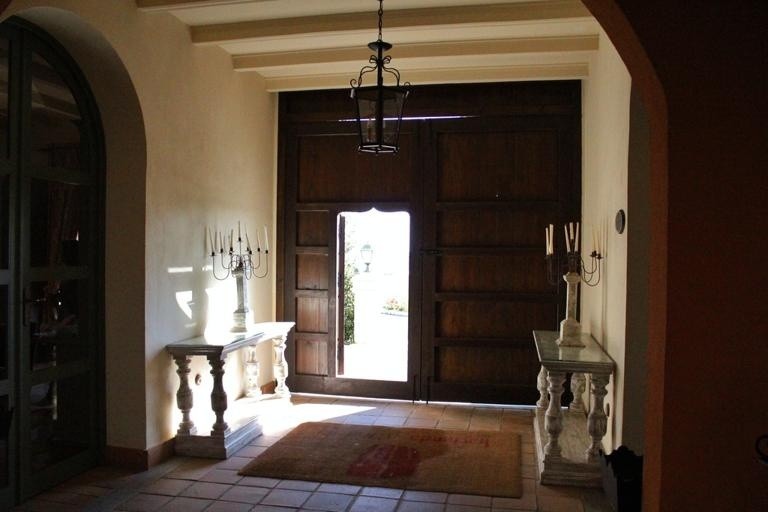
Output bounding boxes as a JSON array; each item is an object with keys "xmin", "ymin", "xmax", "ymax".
[{"xmin": 346, "ymin": 2, "xmax": 415, "ymax": 157}]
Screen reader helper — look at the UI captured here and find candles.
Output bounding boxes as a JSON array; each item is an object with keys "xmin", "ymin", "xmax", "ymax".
[
  {"xmin": 543, "ymin": 217, "xmax": 608, "ymax": 259},
  {"xmin": 206, "ymin": 220, "xmax": 270, "ymax": 257}
]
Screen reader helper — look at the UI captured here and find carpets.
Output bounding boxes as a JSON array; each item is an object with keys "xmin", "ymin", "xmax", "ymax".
[{"xmin": 236, "ymin": 420, "xmax": 524, "ymax": 499}]
